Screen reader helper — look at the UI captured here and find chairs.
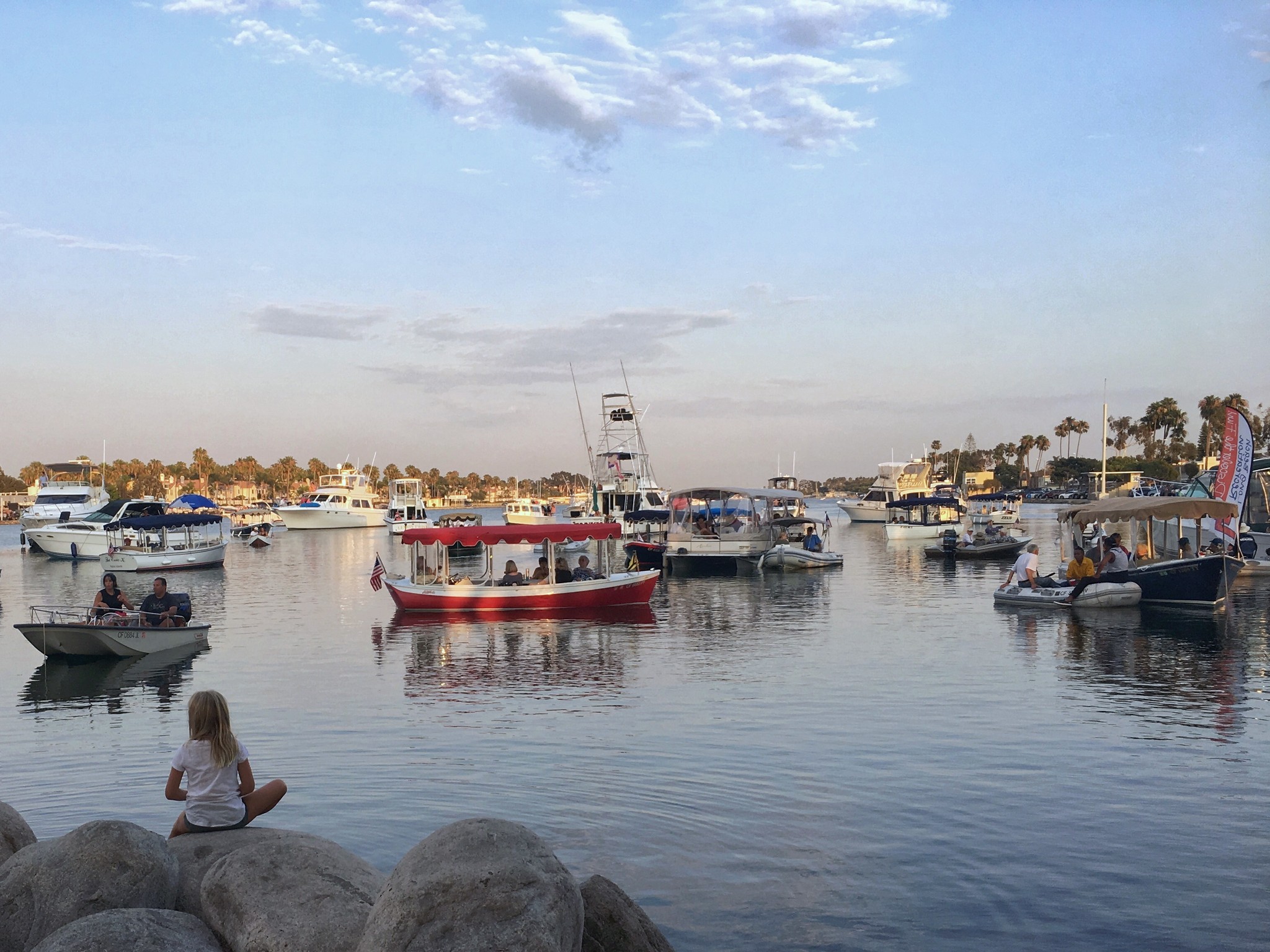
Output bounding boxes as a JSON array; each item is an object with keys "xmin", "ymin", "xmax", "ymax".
[{"xmin": 714, "ymin": 526, "xmax": 745, "ymax": 535}]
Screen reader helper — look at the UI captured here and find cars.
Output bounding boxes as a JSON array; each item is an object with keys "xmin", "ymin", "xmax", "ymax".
[
  {"xmin": 1128, "ymin": 478, "xmax": 1209, "ymax": 499},
  {"xmin": 994, "ymin": 487, "xmax": 1089, "ymax": 499}
]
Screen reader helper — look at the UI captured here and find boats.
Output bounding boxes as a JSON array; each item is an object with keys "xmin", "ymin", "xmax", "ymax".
[
  {"xmin": 929, "ymin": 442, "xmax": 969, "ymax": 517},
  {"xmin": 17, "ymin": 437, "xmax": 110, "ymax": 547},
  {"xmin": 762, "ymin": 517, "xmax": 844, "ymax": 570},
  {"xmin": 533, "ymin": 538, "xmax": 592, "ymax": 553},
  {"xmin": 382, "ymin": 478, "xmax": 434, "ymax": 536},
  {"xmin": 923, "ymin": 526, "xmax": 1034, "ymax": 558},
  {"xmin": 13, "ymin": 605, "xmax": 212, "ymax": 659},
  {"xmin": 836, "ymin": 442, "xmax": 955, "ymax": 524},
  {"xmin": 881, "ymin": 496, "xmax": 965, "ymax": 540},
  {"xmin": 993, "ymin": 579, "xmax": 1142, "ymax": 607},
  {"xmin": 271, "ymin": 451, "xmax": 389, "ymax": 530},
  {"xmin": 23, "ymin": 493, "xmax": 170, "ymax": 561},
  {"xmin": 1055, "ymin": 497, "xmax": 1247, "ymax": 605},
  {"xmin": 1129, "ymin": 457, "xmax": 1270, "ymax": 561},
  {"xmin": 370, "ymin": 521, "xmax": 661, "ymax": 612},
  {"xmin": 968, "ymin": 489, "xmax": 1024, "ymax": 525},
  {"xmin": 219, "ymin": 501, "xmax": 286, "ymax": 549},
  {"xmin": 1234, "ymin": 555, "xmax": 1270, "ymax": 576},
  {"xmin": 501, "ymin": 355, "xmax": 810, "ymax": 578},
  {"xmin": 433, "ymin": 513, "xmax": 487, "ymax": 557},
  {"xmin": 98, "ymin": 512, "xmax": 230, "ymax": 572}
]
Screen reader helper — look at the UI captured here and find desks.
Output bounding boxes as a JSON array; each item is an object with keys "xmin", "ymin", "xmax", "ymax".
[{"xmin": 522, "ymin": 578, "xmax": 544, "ymax": 584}]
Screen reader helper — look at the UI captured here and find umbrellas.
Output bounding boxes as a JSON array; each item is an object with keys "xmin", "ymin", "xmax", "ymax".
[{"xmin": 592, "ymin": 482, "xmax": 598, "ymax": 516}]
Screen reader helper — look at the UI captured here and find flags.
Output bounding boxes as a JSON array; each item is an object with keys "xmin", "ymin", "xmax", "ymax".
[
  {"xmin": 108, "ymin": 542, "xmax": 120, "ymax": 558},
  {"xmin": 824, "ymin": 514, "xmax": 832, "ymax": 530},
  {"xmin": 637, "ymin": 530, "xmax": 644, "ymax": 542},
  {"xmin": 370, "ymin": 555, "xmax": 385, "ymax": 592},
  {"xmin": 1012, "ymin": 517, "xmax": 1020, "ymax": 528},
  {"xmin": 1214, "ymin": 405, "xmax": 1254, "ymax": 545},
  {"xmin": 608, "ymin": 453, "xmax": 624, "ymax": 479}
]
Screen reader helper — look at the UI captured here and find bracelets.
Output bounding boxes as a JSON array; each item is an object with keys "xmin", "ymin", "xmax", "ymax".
[
  {"xmin": 1006, "ymin": 580, "xmax": 1011, "ymax": 583},
  {"xmin": 538, "ymin": 582, "xmax": 539, "ymax": 585}
]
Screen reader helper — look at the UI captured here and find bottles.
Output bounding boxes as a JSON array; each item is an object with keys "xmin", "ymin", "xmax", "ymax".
[{"xmin": 526, "ymin": 569, "xmax": 529, "ymax": 580}]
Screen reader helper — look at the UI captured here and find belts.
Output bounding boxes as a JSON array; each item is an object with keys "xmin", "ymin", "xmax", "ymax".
[{"xmin": 1018, "ymin": 579, "xmax": 1030, "ymax": 583}]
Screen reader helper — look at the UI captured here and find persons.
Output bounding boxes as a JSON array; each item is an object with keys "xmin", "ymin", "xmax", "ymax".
[
  {"xmin": 936, "ymin": 520, "xmax": 1018, "ymax": 549},
  {"xmin": 90, "ymin": 573, "xmax": 135, "ymax": 626},
  {"xmin": 130, "ymin": 577, "xmax": 178, "ymax": 627},
  {"xmin": 892, "ymin": 513, "xmax": 943, "ymax": 524},
  {"xmin": 38, "ymin": 471, "xmax": 49, "ymax": 490},
  {"xmin": 165, "ymin": 690, "xmax": 288, "ymax": 839},
  {"xmin": 124, "ymin": 536, "xmax": 154, "ymax": 553},
  {"xmin": 982, "ymin": 505, "xmax": 1006, "ymax": 515},
  {"xmin": 776, "ymin": 526, "xmax": 823, "ymax": 553},
  {"xmin": 235, "ymin": 514, "xmax": 267, "ymax": 538},
  {"xmin": 1178, "ymin": 523, "xmax": 1258, "ymax": 560},
  {"xmin": 999, "ymin": 533, "xmax": 1148, "ymax": 604},
  {"xmin": 416, "ymin": 551, "xmax": 596, "ymax": 585},
  {"xmin": 279, "ymin": 478, "xmax": 337, "ymax": 506},
  {"xmin": 541, "ymin": 503, "xmax": 556, "ymax": 516},
  {"xmin": 685, "ymin": 515, "xmax": 718, "ymax": 539},
  {"xmin": 394, "ymin": 511, "xmax": 402, "ymax": 521},
  {"xmin": 593, "ymin": 511, "xmax": 600, "ymax": 517}
]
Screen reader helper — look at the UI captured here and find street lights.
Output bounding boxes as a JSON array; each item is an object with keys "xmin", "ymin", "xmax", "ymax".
[{"xmin": 1035, "ymin": 458, "xmax": 1046, "ymax": 488}]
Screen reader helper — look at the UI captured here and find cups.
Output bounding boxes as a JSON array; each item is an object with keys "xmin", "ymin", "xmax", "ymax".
[
  {"xmin": 1199, "ymin": 552, "xmax": 1203, "ymax": 555},
  {"xmin": 522, "ymin": 575, "xmax": 524, "ymax": 581},
  {"xmin": 1207, "ymin": 550, "xmax": 1210, "ymax": 555},
  {"xmin": 1069, "ymin": 579, "xmax": 1076, "ymax": 586}
]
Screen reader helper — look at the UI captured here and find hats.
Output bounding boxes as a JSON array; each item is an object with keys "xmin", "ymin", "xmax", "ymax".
[
  {"xmin": 987, "ymin": 519, "xmax": 994, "ymax": 524},
  {"xmin": 725, "ymin": 515, "xmax": 737, "ymax": 526},
  {"xmin": 1001, "ymin": 528, "xmax": 1008, "ymax": 533},
  {"xmin": 1136, "ymin": 543, "xmax": 1148, "ymax": 556},
  {"xmin": 1239, "ymin": 523, "xmax": 1250, "ymax": 533},
  {"xmin": 973, "ymin": 532, "xmax": 986, "ymax": 539},
  {"xmin": 1209, "ymin": 538, "xmax": 1223, "ymax": 544},
  {"xmin": 967, "ymin": 527, "xmax": 974, "ymax": 532},
  {"xmin": 1177, "ymin": 537, "xmax": 1189, "ymax": 543}
]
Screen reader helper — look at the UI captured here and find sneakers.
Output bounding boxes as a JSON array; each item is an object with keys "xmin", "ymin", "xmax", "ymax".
[{"xmin": 1054, "ymin": 599, "xmax": 1072, "ymax": 606}]
